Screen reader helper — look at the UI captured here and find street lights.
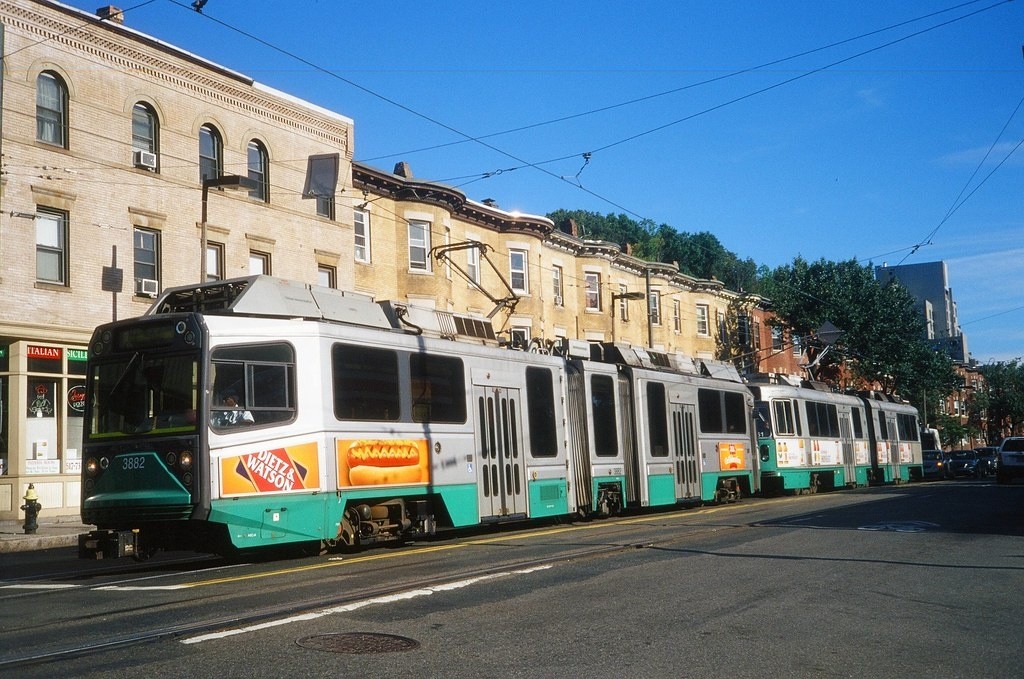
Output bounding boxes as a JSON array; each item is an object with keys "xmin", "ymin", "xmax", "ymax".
[
  {"xmin": 200, "ymin": 171, "xmax": 257, "ymax": 283},
  {"xmin": 612, "ymin": 291, "xmax": 646, "ymax": 341}
]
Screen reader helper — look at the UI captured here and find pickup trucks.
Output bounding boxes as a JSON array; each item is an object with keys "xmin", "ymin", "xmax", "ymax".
[{"xmin": 999, "ymin": 437, "xmax": 1024, "ymax": 484}]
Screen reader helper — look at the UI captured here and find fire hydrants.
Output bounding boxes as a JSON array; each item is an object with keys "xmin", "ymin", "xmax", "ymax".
[{"xmin": 19, "ymin": 484, "xmax": 46, "ymax": 534}]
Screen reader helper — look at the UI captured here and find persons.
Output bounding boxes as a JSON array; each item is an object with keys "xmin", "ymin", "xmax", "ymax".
[
  {"xmin": 210, "ymin": 386, "xmax": 255, "ymax": 427},
  {"xmin": 762, "ymin": 421, "xmax": 770, "ymax": 436},
  {"xmin": 809, "ymin": 416, "xmax": 818, "ymax": 436}
]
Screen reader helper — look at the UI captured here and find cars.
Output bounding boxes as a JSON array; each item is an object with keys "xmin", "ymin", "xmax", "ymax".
[
  {"xmin": 922, "ymin": 449, "xmax": 945, "ymax": 481},
  {"xmin": 974, "ymin": 447, "xmax": 999, "ymax": 475},
  {"xmin": 947, "ymin": 450, "xmax": 984, "ymax": 481}
]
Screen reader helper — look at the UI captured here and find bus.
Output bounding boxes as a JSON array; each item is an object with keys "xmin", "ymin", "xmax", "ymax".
[
  {"xmin": 748, "ymin": 332, "xmax": 925, "ymax": 495},
  {"xmin": 80, "ymin": 239, "xmax": 767, "ymax": 566}
]
